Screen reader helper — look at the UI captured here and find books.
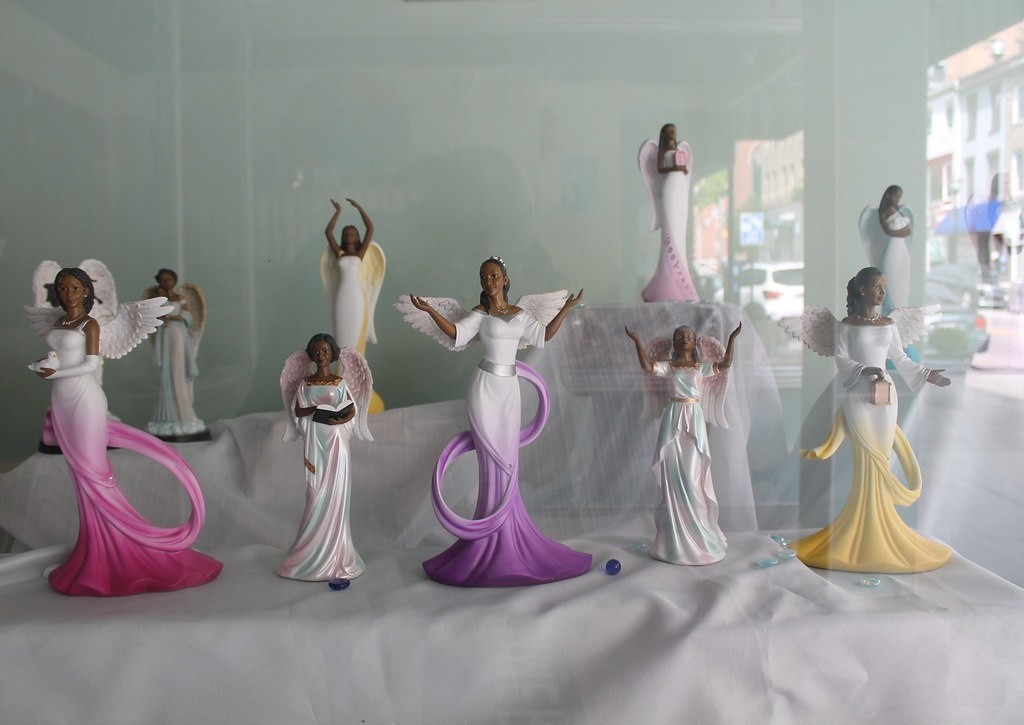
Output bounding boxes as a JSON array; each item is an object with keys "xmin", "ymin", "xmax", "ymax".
[{"xmin": 312, "ymin": 400, "xmax": 354, "ymax": 425}]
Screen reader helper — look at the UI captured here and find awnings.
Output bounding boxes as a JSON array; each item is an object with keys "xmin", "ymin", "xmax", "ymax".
[{"xmin": 935, "ymin": 201, "xmax": 1013, "ymax": 235}]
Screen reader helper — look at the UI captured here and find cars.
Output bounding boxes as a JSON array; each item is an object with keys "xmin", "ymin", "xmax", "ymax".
[
  {"xmin": 920, "ymin": 281, "xmax": 1007, "ymax": 375},
  {"xmin": 710, "ymin": 258, "xmax": 805, "ymax": 328}
]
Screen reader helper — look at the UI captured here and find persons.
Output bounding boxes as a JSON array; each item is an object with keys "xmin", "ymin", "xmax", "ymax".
[
  {"xmin": 881, "ymin": 187, "xmax": 912, "ymax": 308},
  {"xmin": 638, "ymin": 124, "xmax": 700, "ymax": 302},
  {"xmin": 393, "ymin": 256, "xmax": 593, "ymax": 588},
  {"xmin": 777, "ymin": 267, "xmax": 952, "ymax": 573},
  {"xmin": 321, "ymin": 199, "xmax": 386, "ymax": 415},
  {"xmin": 625, "ymin": 321, "xmax": 743, "ymax": 566},
  {"xmin": 22, "ymin": 258, "xmax": 222, "ymax": 596},
  {"xmin": 275, "ymin": 333, "xmax": 372, "ymax": 585}
]
